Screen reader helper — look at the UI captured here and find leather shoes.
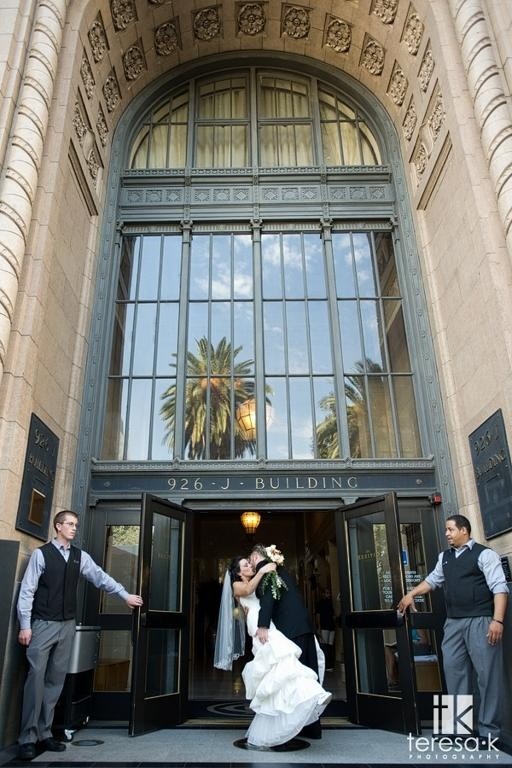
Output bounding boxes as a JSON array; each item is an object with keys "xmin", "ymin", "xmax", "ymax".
[
  {"xmin": 432, "ymin": 734, "xmax": 477, "ymax": 745},
  {"xmin": 480, "ymin": 738, "xmax": 500, "ymax": 750},
  {"xmin": 19, "ymin": 743, "xmax": 37, "ymax": 759},
  {"xmin": 37, "ymin": 737, "xmax": 66, "ymax": 751}
]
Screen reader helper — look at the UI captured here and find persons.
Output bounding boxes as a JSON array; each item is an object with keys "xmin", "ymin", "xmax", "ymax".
[
  {"xmin": 228, "ymin": 556, "xmax": 332, "ymax": 747},
  {"xmin": 247, "ymin": 544, "xmax": 322, "ymax": 740},
  {"xmin": 381, "ymin": 628, "xmax": 402, "ymax": 694},
  {"xmin": 16, "ymin": 509, "xmax": 146, "ymax": 760},
  {"xmin": 412, "ymin": 628, "xmax": 429, "ymax": 647},
  {"xmin": 397, "ymin": 515, "xmax": 509, "ymax": 750},
  {"xmin": 316, "ymin": 586, "xmax": 336, "ymax": 672}
]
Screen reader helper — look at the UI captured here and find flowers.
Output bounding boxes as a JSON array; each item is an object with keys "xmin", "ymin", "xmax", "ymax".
[{"xmin": 260, "ymin": 545, "xmax": 289, "ymax": 601}]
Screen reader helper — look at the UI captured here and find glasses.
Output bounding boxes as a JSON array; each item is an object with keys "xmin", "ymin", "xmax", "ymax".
[{"xmin": 62, "ymin": 522, "xmax": 80, "ymax": 528}]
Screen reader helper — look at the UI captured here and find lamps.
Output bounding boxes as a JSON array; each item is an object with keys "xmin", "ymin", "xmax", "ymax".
[
  {"xmin": 241, "ymin": 511, "xmax": 261, "ymax": 535},
  {"xmin": 236, "ymin": 394, "xmax": 273, "ymax": 442}
]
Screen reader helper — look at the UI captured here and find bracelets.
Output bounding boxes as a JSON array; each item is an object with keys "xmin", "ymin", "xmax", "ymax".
[{"xmin": 490, "ymin": 618, "xmax": 504, "ymax": 626}]
